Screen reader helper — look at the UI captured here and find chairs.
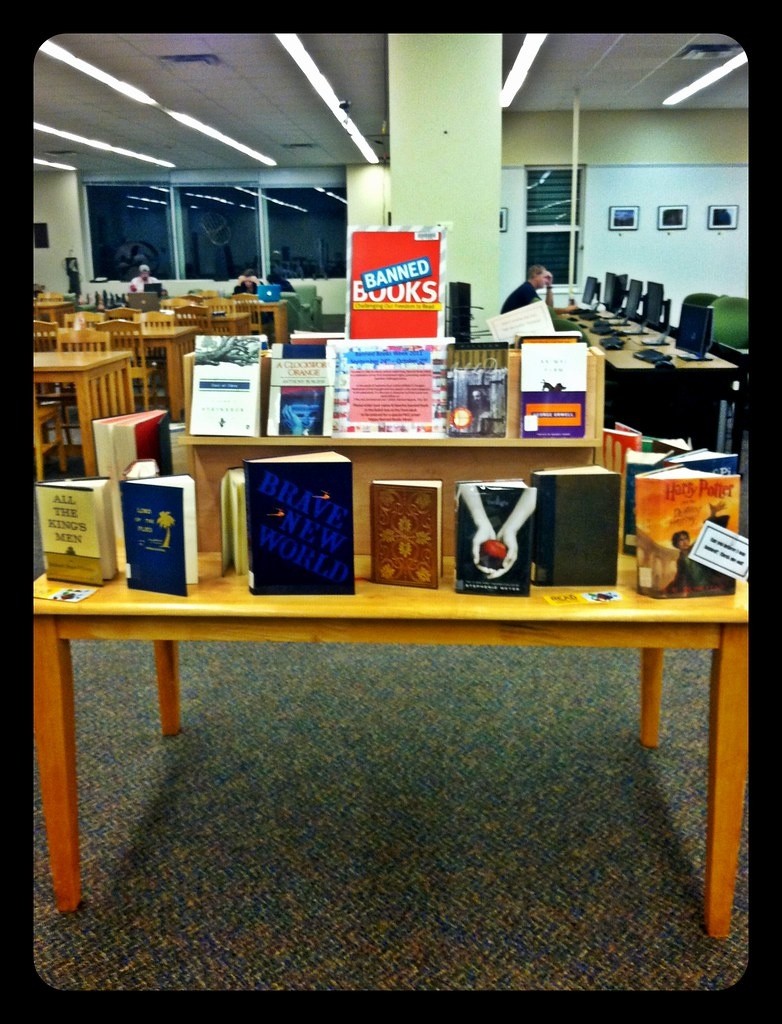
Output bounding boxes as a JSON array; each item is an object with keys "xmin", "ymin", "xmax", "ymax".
[
  {"xmin": 35, "ymin": 291, "xmax": 265, "ymax": 483},
  {"xmin": 683, "ymin": 293, "xmax": 718, "ymax": 308},
  {"xmin": 532, "ymin": 297, "xmax": 593, "ymax": 347},
  {"xmin": 707, "ymin": 297, "xmax": 749, "ymax": 350}
]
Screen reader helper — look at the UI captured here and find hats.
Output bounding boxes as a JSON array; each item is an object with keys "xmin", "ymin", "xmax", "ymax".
[
  {"xmin": 139, "ymin": 264, "xmax": 149, "ymax": 272},
  {"xmin": 244, "ymin": 269, "xmax": 256, "ymax": 276}
]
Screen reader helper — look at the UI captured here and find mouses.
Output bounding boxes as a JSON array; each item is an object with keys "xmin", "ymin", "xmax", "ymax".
[
  {"xmin": 655, "ymin": 359, "xmax": 675, "ymax": 368},
  {"xmin": 607, "ymin": 343, "xmax": 621, "ymax": 350},
  {"xmin": 612, "ymin": 331, "xmax": 625, "ymax": 336}
]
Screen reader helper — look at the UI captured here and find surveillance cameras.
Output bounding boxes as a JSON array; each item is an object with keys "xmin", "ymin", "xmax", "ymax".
[{"xmin": 338, "ymin": 101, "xmax": 351, "ymax": 109}]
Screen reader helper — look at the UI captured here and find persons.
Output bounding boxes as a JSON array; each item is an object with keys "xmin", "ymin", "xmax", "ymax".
[
  {"xmin": 280, "ymin": 392, "xmax": 320, "ymax": 436},
  {"xmin": 232, "ymin": 269, "xmax": 262, "ymax": 295},
  {"xmin": 459, "ymin": 485, "xmax": 537, "ymax": 579},
  {"xmin": 130, "ymin": 265, "xmax": 159, "ymax": 293},
  {"xmin": 267, "ymin": 270, "xmax": 294, "ymax": 292},
  {"xmin": 501, "ymin": 265, "xmax": 576, "ymax": 315}
]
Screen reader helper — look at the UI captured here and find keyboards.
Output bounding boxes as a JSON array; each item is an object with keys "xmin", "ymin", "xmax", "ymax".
[
  {"xmin": 599, "ymin": 337, "xmax": 624, "ymax": 345},
  {"xmin": 632, "ymin": 348, "xmax": 672, "ymax": 362},
  {"xmin": 594, "ymin": 319, "xmax": 610, "ymax": 327},
  {"xmin": 581, "ymin": 312, "xmax": 600, "ymax": 320},
  {"xmin": 589, "ymin": 326, "xmax": 610, "ymax": 334},
  {"xmin": 568, "ymin": 308, "xmax": 584, "ymax": 316}
]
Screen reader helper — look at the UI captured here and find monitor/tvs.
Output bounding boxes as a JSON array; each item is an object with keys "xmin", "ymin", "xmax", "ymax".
[{"xmin": 580, "ymin": 273, "xmax": 716, "ymax": 361}]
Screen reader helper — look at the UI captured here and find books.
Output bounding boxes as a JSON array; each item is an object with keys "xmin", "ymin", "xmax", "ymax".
[
  {"xmin": 190, "ymin": 334, "xmax": 262, "ymax": 437},
  {"xmin": 33, "ymin": 476, "xmax": 118, "ymax": 585},
  {"xmin": 453, "ymin": 479, "xmax": 536, "ymax": 597},
  {"xmin": 267, "ymin": 344, "xmax": 335, "ymax": 438},
  {"xmin": 91, "ymin": 409, "xmax": 173, "ymax": 475},
  {"xmin": 123, "ymin": 458, "xmax": 158, "ymax": 480},
  {"xmin": 219, "ymin": 466, "xmax": 249, "ymax": 578},
  {"xmin": 291, "ymin": 330, "xmax": 345, "ymax": 344},
  {"xmin": 243, "ymin": 450, "xmax": 356, "ymax": 596},
  {"xmin": 119, "ymin": 474, "xmax": 198, "ymax": 598},
  {"xmin": 516, "ymin": 330, "xmax": 582, "ymax": 349},
  {"xmin": 370, "ymin": 478, "xmax": 444, "ymax": 589},
  {"xmin": 451, "ymin": 340, "xmax": 740, "ymax": 599}
]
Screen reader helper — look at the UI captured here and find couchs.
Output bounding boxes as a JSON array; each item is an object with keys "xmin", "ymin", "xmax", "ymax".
[{"xmin": 280, "ymin": 284, "xmax": 323, "ymax": 335}]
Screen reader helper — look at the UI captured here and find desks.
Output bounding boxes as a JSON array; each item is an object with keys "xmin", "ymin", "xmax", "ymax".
[
  {"xmin": 558, "ymin": 311, "xmax": 741, "ymax": 451},
  {"xmin": 174, "ymin": 312, "xmax": 252, "ymax": 335},
  {"xmin": 34, "ymin": 351, "xmax": 133, "ymax": 476},
  {"xmin": 34, "ymin": 325, "xmax": 197, "ymax": 422},
  {"xmin": 34, "ymin": 301, "xmax": 75, "ymax": 322},
  {"xmin": 202, "ymin": 299, "xmax": 289, "ymax": 344},
  {"xmin": 33, "ymin": 554, "xmax": 749, "ymax": 940}
]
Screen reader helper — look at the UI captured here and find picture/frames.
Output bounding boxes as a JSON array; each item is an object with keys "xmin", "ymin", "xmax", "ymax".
[
  {"xmin": 499, "ymin": 207, "xmax": 508, "ymax": 233},
  {"xmin": 707, "ymin": 205, "xmax": 739, "ymax": 230},
  {"xmin": 608, "ymin": 206, "xmax": 639, "ymax": 230},
  {"xmin": 657, "ymin": 205, "xmax": 688, "ymax": 230}
]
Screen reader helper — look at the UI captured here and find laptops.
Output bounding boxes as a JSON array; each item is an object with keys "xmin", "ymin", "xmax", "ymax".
[
  {"xmin": 254, "ymin": 285, "xmax": 281, "ymax": 302},
  {"xmin": 128, "ymin": 292, "xmax": 161, "ymax": 312},
  {"xmin": 144, "ymin": 283, "xmax": 163, "ymax": 298}
]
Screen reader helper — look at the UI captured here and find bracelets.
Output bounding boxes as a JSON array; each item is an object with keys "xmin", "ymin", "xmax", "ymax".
[{"xmin": 547, "ymin": 286, "xmax": 551, "ymax": 288}]
